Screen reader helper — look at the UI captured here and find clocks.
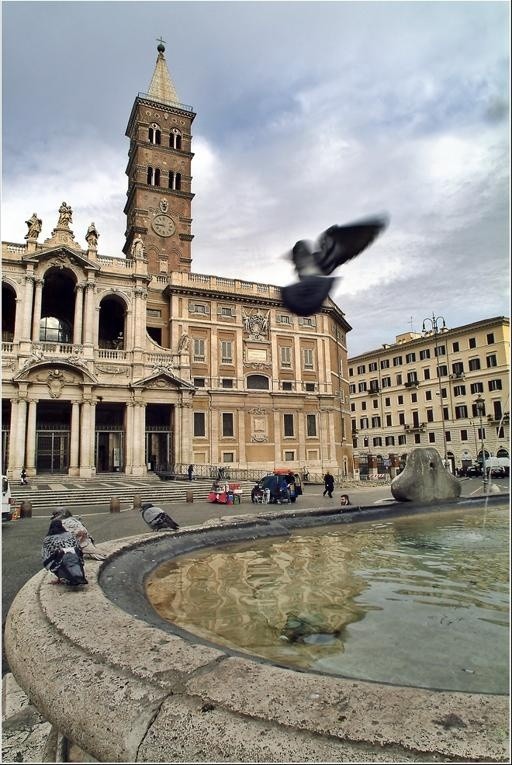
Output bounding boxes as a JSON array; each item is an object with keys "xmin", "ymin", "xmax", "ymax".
[{"xmin": 152, "ymin": 213, "xmax": 175, "ymax": 237}]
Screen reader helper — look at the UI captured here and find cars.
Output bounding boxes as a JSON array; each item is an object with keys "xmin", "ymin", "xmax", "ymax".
[
  {"xmin": 252, "ymin": 474, "xmax": 302, "ymax": 504},
  {"xmin": 460, "ymin": 466, "xmax": 505, "ymax": 479}
]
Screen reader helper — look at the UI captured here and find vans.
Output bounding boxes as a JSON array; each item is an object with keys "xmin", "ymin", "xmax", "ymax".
[{"xmin": 1, "ymin": 476, "xmax": 12, "ymax": 521}]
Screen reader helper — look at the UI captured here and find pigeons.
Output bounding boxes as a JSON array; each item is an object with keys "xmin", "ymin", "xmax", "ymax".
[
  {"xmin": 39, "ymin": 517, "xmax": 90, "ymax": 587},
  {"xmin": 277, "ymin": 215, "xmax": 389, "ymax": 317},
  {"xmin": 50, "ymin": 507, "xmax": 106, "ymax": 562},
  {"xmin": 137, "ymin": 502, "xmax": 179, "ymax": 535}
]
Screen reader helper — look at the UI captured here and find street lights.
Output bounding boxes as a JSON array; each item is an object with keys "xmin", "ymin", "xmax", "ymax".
[
  {"xmin": 475, "ymin": 395, "xmax": 488, "ymax": 493},
  {"xmin": 422, "ymin": 313, "xmax": 449, "ymax": 462}
]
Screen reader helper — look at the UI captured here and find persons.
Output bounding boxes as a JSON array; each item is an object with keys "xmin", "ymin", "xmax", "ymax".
[
  {"xmin": 188, "ymin": 464, "xmax": 195, "ymax": 480},
  {"xmin": 210, "ymin": 478, "xmax": 220, "ymax": 492},
  {"xmin": 285, "ymin": 472, "xmax": 295, "ymax": 504},
  {"xmin": 20, "ymin": 466, "xmax": 28, "ymax": 485},
  {"xmin": 322, "ymin": 471, "xmax": 334, "ymax": 498},
  {"xmin": 340, "ymin": 494, "xmax": 351, "ymax": 506}
]
